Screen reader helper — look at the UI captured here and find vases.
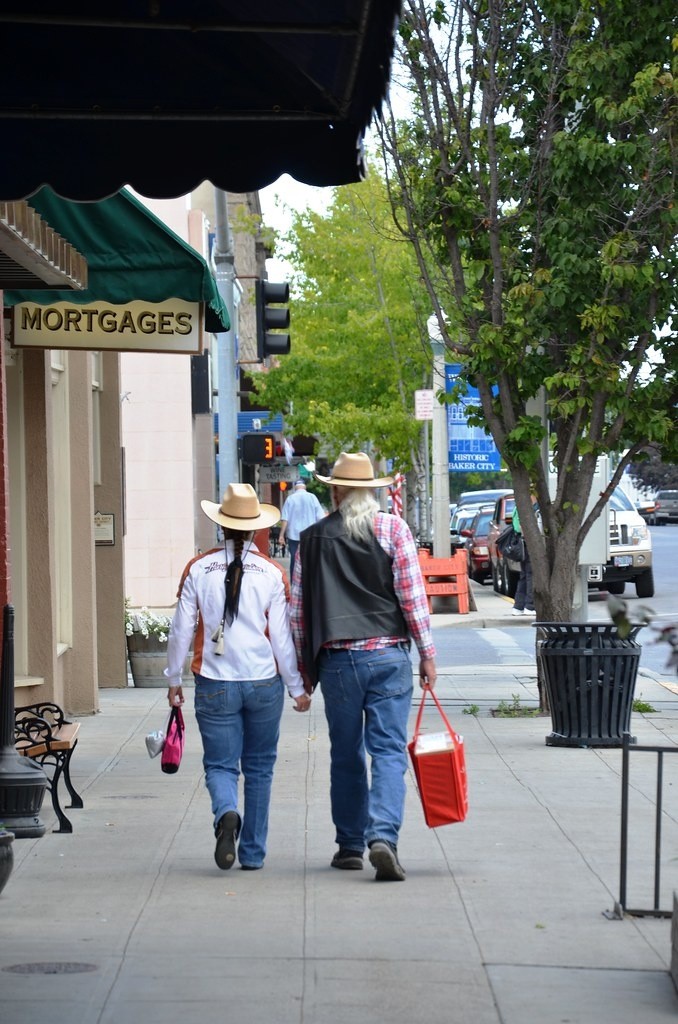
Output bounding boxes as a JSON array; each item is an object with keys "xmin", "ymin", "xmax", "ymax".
[{"xmin": 126, "ymin": 631, "xmax": 193, "ymax": 687}]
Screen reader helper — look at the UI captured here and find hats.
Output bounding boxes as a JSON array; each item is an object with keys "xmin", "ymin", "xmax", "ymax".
[
  {"xmin": 295, "ymin": 479, "xmax": 305, "ymax": 486},
  {"xmin": 201, "ymin": 483, "xmax": 280, "ymax": 531},
  {"xmin": 315, "ymin": 452, "xmax": 394, "ymax": 487}
]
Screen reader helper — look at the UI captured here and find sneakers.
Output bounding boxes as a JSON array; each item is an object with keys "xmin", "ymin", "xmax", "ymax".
[
  {"xmin": 524, "ymin": 608, "xmax": 536, "ymax": 616},
  {"xmin": 512, "ymin": 608, "xmax": 525, "ymax": 616}
]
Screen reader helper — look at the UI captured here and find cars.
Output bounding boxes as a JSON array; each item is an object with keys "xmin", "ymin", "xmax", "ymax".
[
  {"xmin": 450, "ymin": 485, "xmax": 656, "ymax": 599},
  {"xmin": 651, "ymin": 490, "xmax": 678, "ymax": 527}
]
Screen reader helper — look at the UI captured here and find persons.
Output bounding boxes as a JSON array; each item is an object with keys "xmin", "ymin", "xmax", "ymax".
[
  {"xmin": 291, "ymin": 450, "xmax": 438, "ymax": 879},
  {"xmin": 511, "ymin": 490, "xmax": 537, "ymax": 616},
  {"xmin": 163, "ymin": 483, "xmax": 309, "ymax": 864},
  {"xmin": 278, "ymin": 479, "xmax": 326, "ymax": 585}
]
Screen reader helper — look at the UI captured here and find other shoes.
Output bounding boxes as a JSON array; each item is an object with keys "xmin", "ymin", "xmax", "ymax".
[
  {"xmin": 242, "ymin": 864, "xmax": 263, "ymax": 870},
  {"xmin": 214, "ymin": 810, "xmax": 238, "ymax": 870},
  {"xmin": 369, "ymin": 840, "xmax": 406, "ymax": 880},
  {"xmin": 331, "ymin": 848, "xmax": 365, "ymax": 870}
]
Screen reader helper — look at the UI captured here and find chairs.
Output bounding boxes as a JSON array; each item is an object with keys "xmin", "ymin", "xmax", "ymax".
[{"xmin": 478, "ymin": 522, "xmax": 490, "ymax": 535}]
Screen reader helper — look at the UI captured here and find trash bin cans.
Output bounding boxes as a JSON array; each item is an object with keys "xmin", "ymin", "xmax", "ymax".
[{"xmin": 530, "ymin": 621, "xmax": 649, "ymax": 746}]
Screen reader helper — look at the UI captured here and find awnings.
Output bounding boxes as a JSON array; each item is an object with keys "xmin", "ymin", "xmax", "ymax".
[
  {"xmin": 273, "ymin": 462, "xmax": 313, "ymax": 484},
  {"xmin": 0, "ymin": 0, "xmax": 399, "ymax": 331}
]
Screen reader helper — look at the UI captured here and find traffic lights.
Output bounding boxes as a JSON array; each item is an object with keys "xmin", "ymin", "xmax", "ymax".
[
  {"xmin": 241, "ymin": 433, "xmax": 277, "ymax": 467},
  {"xmin": 254, "ymin": 277, "xmax": 294, "ymax": 361}
]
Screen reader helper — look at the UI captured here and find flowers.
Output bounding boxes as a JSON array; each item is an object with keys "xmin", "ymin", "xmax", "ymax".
[{"xmin": 124, "ymin": 596, "xmax": 199, "ymax": 642}]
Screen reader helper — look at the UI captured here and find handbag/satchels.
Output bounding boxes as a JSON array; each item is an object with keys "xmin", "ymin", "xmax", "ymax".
[
  {"xmin": 162, "ymin": 706, "xmax": 186, "ymax": 774},
  {"xmin": 408, "ymin": 683, "xmax": 467, "ymax": 828},
  {"xmin": 495, "ymin": 527, "xmax": 526, "ymax": 563}
]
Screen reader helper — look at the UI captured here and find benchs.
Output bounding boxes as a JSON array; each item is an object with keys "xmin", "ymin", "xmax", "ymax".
[{"xmin": 15, "ymin": 702, "xmax": 83, "ymax": 833}]
